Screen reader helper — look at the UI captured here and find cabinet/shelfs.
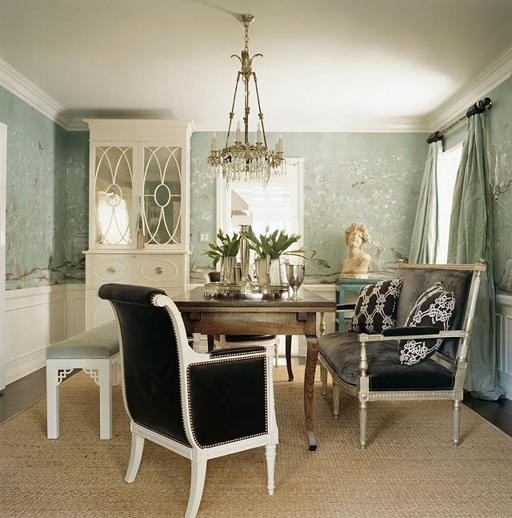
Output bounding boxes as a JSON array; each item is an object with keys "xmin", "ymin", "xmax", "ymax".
[
  {"xmin": 78, "ymin": 116, "xmax": 200, "ymax": 334},
  {"xmin": 293, "ymin": 284, "xmax": 337, "ymax": 358}
]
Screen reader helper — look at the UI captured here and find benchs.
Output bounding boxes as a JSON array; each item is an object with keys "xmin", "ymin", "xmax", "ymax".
[
  {"xmin": 43, "ymin": 319, "xmax": 122, "ymax": 442},
  {"xmin": 311, "ymin": 260, "xmax": 487, "ymax": 452}
]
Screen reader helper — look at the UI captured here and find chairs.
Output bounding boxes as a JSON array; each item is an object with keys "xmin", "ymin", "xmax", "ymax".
[{"xmin": 95, "ymin": 282, "xmax": 280, "ymax": 516}]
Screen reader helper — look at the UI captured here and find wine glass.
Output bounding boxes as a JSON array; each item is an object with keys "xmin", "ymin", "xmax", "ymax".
[{"xmin": 285, "ymin": 258, "xmax": 306, "ymax": 301}]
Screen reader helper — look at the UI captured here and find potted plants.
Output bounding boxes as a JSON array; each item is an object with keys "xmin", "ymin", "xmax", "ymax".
[
  {"xmin": 204, "ymin": 232, "xmax": 243, "ymax": 289},
  {"xmin": 241, "ymin": 227, "xmax": 318, "ymax": 288}
]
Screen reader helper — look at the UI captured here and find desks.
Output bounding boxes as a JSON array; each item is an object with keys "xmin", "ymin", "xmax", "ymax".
[{"xmin": 169, "ymin": 279, "xmax": 340, "ymax": 454}]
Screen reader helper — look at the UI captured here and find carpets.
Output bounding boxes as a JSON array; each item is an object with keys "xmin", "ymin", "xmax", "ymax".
[{"xmin": 1, "ymin": 363, "xmax": 511, "ymax": 518}]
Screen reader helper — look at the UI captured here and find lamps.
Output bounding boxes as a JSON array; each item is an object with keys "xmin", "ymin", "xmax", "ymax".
[
  {"xmin": 202, "ymin": 13, "xmax": 289, "ymax": 188},
  {"xmin": 484, "ymin": 150, "xmax": 512, "ymax": 200}
]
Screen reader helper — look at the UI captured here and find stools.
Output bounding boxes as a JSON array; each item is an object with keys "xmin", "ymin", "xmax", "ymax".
[{"xmin": 211, "ymin": 331, "xmax": 279, "ymax": 366}]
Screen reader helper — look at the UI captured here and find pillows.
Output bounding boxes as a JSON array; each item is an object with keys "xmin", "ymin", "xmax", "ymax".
[
  {"xmin": 397, "ymin": 280, "xmax": 456, "ymax": 367},
  {"xmin": 349, "ymin": 276, "xmax": 403, "ymax": 338}
]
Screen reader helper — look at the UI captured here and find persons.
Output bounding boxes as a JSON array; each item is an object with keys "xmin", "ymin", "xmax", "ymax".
[{"xmin": 341, "ymin": 224, "xmax": 370, "ymax": 275}]
